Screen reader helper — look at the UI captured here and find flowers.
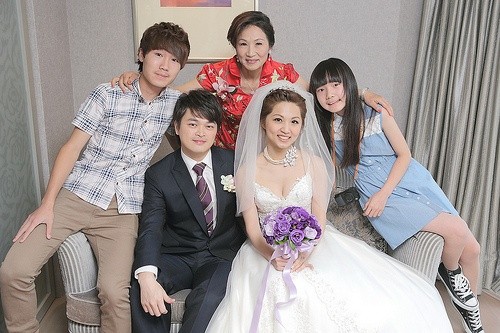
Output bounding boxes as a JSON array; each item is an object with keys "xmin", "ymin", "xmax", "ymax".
[
  {"xmin": 212, "ymin": 77, "xmax": 239, "ymax": 102},
  {"xmin": 262, "ymin": 205, "xmax": 322, "ymax": 260},
  {"xmin": 221, "ymin": 174, "xmax": 236, "ymax": 193}
]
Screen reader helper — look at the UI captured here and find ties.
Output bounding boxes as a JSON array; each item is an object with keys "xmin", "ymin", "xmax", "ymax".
[{"xmin": 192, "ymin": 162, "xmax": 214, "ymax": 238}]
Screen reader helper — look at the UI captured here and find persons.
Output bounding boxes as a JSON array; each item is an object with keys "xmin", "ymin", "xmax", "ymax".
[
  {"xmin": 110, "ymin": 10, "xmax": 394, "ymax": 149},
  {"xmin": 309, "ymin": 58, "xmax": 484, "ymax": 333},
  {"xmin": 236, "ymin": 90, "xmax": 357, "ymax": 333},
  {"xmin": 130, "ymin": 90, "xmax": 246, "ymax": 333},
  {"xmin": 0, "ymin": 22, "xmax": 190, "ymax": 333}
]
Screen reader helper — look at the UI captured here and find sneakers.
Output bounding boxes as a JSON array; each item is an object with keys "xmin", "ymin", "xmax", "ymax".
[
  {"xmin": 437, "ymin": 261, "xmax": 480, "ymax": 312},
  {"xmin": 450, "ymin": 298, "xmax": 486, "ymax": 333}
]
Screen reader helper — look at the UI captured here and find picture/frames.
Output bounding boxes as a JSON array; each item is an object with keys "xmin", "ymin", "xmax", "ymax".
[{"xmin": 131, "ymin": 0, "xmax": 259, "ymax": 63}]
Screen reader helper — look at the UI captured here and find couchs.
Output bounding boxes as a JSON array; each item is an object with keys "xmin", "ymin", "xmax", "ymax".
[{"xmin": 57, "ymin": 166, "xmax": 445, "ymax": 333}]
